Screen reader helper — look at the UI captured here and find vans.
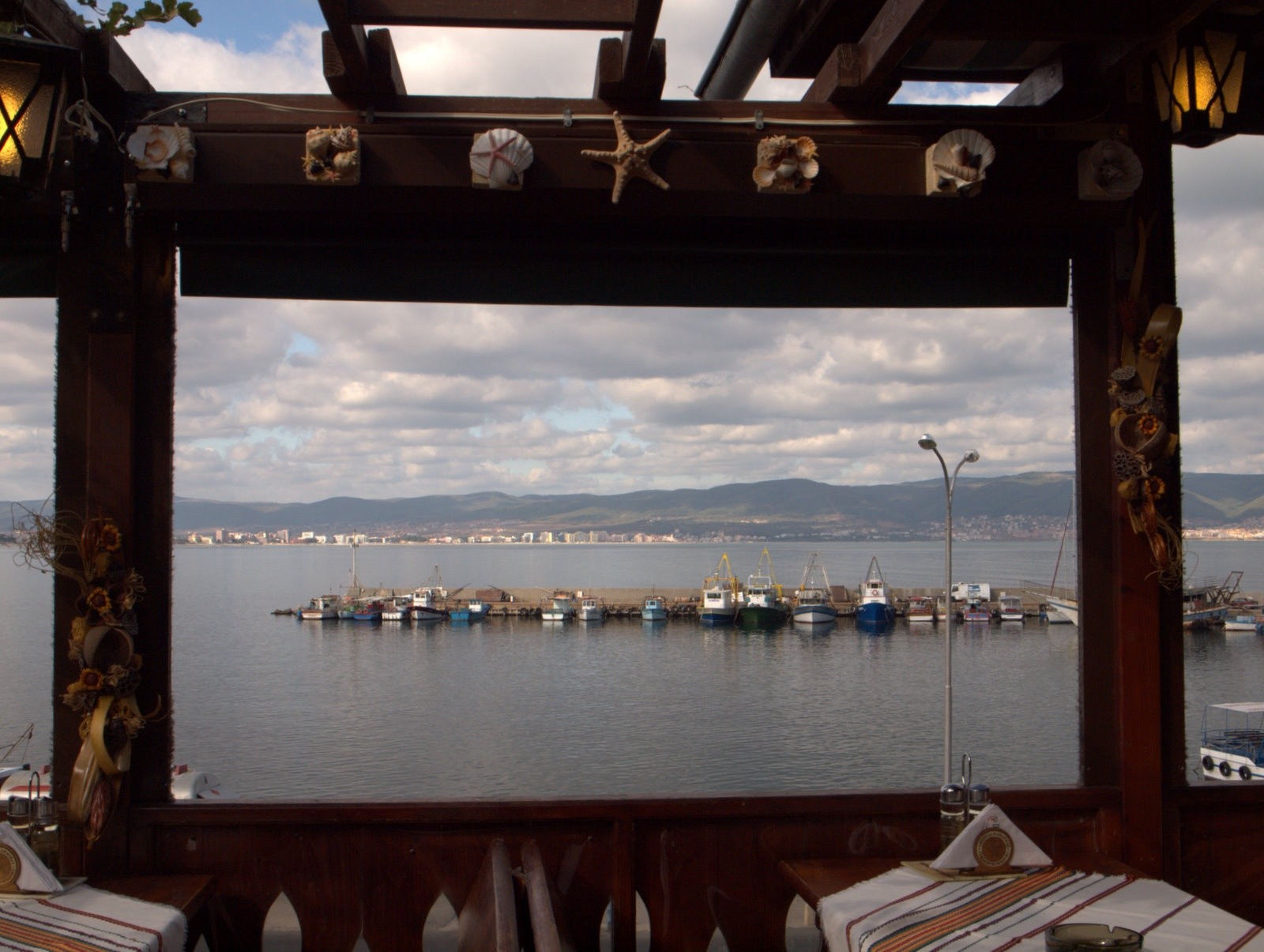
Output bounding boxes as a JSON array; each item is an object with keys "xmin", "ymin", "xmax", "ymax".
[{"xmin": 943, "ymin": 581, "xmax": 991, "ymax": 603}]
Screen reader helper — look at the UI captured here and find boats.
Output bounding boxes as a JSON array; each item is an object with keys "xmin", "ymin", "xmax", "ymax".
[
  {"xmin": 1199, "ymin": 703, "xmax": 1264, "ymax": 781},
  {"xmin": 790, "ymin": 551, "xmax": 838, "ymax": 624},
  {"xmin": 540, "ymin": 596, "xmax": 575, "ymax": 616},
  {"xmin": 448, "ymin": 598, "xmax": 492, "ymax": 621},
  {"xmin": 695, "ymin": 554, "xmax": 743, "ymax": 627},
  {"xmin": 298, "ymin": 527, "xmax": 450, "ymax": 622},
  {"xmin": 579, "ymin": 582, "xmax": 608, "ymax": 621},
  {"xmin": 734, "ymin": 544, "xmax": 792, "ymax": 630},
  {"xmin": 955, "ymin": 598, "xmax": 993, "ymax": 623},
  {"xmin": 996, "ymin": 592, "xmax": 1025, "ymax": 622},
  {"xmin": 853, "ymin": 556, "xmax": 898, "ymax": 624},
  {"xmin": 902, "ymin": 596, "xmax": 936, "ymax": 623},
  {"xmin": 1021, "ymin": 500, "xmax": 1264, "ymax": 632},
  {"xmin": 640, "ymin": 584, "xmax": 670, "ymax": 621}
]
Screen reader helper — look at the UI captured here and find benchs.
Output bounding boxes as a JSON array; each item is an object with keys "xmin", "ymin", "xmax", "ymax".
[{"xmin": 447, "ymin": 841, "xmax": 567, "ymax": 952}]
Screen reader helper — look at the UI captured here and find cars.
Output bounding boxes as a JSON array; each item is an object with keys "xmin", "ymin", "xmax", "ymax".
[{"xmin": 1231, "ymin": 597, "xmax": 1259, "ymax": 607}]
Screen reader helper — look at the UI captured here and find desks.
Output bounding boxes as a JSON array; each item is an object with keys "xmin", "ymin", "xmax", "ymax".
[
  {"xmin": 0, "ymin": 874, "xmax": 216, "ymax": 952},
  {"xmin": 781, "ymin": 859, "xmax": 1264, "ymax": 952}
]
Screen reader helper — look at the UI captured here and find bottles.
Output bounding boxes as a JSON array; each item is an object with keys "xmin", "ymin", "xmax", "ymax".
[
  {"xmin": 968, "ymin": 782, "xmax": 991, "ymax": 822},
  {"xmin": 940, "ymin": 784, "xmax": 965, "ymax": 852},
  {"xmin": 31, "ymin": 797, "xmax": 60, "ymax": 881},
  {"xmin": 9, "ymin": 796, "xmax": 33, "ymax": 848}
]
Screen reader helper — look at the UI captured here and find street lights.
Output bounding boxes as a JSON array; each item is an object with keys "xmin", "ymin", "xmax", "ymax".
[{"xmin": 918, "ymin": 432, "xmax": 979, "ymax": 780}]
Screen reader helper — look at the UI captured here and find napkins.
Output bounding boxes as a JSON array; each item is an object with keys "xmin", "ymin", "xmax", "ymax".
[
  {"xmin": 930, "ymin": 801, "xmax": 1052, "ymax": 866},
  {"xmin": 0, "ymin": 821, "xmax": 63, "ymax": 892}
]
